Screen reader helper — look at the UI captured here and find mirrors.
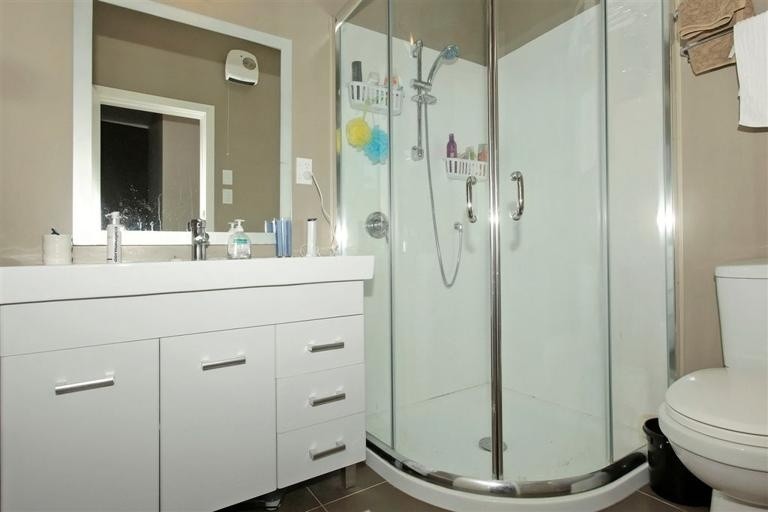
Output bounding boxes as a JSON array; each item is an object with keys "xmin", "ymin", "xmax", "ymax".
[{"xmin": 70, "ymin": 0, "xmax": 293, "ymax": 248}]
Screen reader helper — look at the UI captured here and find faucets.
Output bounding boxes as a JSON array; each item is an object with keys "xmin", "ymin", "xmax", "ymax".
[{"xmin": 194, "ymin": 233, "xmax": 210, "ymax": 246}]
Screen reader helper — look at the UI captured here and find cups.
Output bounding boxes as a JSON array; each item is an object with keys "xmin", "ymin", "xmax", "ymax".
[
  {"xmin": 273, "ymin": 218, "xmax": 293, "ymax": 258},
  {"xmin": 263, "ymin": 218, "xmax": 273, "ymax": 233},
  {"xmin": 42, "ymin": 234, "xmax": 74, "ymax": 265}
]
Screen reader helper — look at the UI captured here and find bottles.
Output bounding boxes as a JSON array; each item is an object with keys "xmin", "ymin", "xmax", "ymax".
[
  {"xmin": 446, "ymin": 132, "xmax": 456, "ymax": 172},
  {"xmin": 105, "ymin": 212, "xmax": 123, "ymax": 264}
]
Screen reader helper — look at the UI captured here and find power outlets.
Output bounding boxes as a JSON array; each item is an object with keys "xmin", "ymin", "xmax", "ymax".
[{"xmin": 296, "ymin": 156, "xmax": 313, "ymax": 186}]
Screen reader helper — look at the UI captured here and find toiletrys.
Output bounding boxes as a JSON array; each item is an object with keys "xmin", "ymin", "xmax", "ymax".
[
  {"xmin": 477, "ymin": 143, "xmax": 488, "ymax": 176},
  {"xmin": 306, "ymin": 217, "xmax": 318, "ymax": 257},
  {"xmin": 464, "ymin": 145, "xmax": 475, "ymax": 173}
]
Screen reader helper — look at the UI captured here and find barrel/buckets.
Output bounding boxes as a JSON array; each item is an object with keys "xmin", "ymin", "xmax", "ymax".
[{"xmin": 656, "ymin": 257, "xmax": 768, "ymax": 510}]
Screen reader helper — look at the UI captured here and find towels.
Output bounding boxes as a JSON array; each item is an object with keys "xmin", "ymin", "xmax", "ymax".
[{"xmin": 674, "ymin": 1, "xmax": 768, "ymax": 128}]
